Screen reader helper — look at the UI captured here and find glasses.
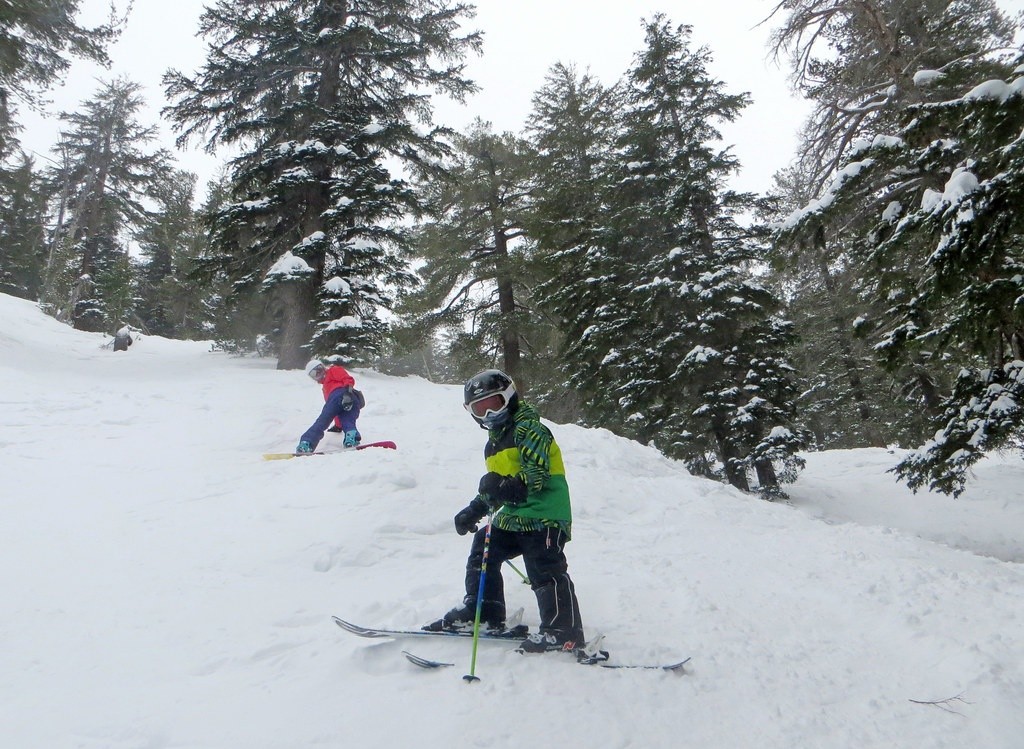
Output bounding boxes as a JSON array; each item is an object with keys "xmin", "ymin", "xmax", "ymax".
[
  {"xmin": 309, "ymin": 365, "xmax": 324, "ymax": 377},
  {"xmin": 466, "ymin": 381, "xmax": 517, "ymax": 420}
]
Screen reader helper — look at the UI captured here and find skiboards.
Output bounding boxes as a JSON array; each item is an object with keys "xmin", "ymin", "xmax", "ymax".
[{"xmin": 330, "ymin": 607, "xmax": 693, "ymax": 672}]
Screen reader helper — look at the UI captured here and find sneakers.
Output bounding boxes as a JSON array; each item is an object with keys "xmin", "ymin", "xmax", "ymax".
[
  {"xmin": 343, "ymin": 428, "xmax": 362, "ymax": 447},
  {"xmin": 296, "ymin": 440, "xmax": 313, "ymax": 454},
  {"xmin": 519, "ymin": 625, "xmax": 587, "ymax": 656},
  {"xmin": 420, "ymin": 594, "xmax": 509, "ymax": 636}
]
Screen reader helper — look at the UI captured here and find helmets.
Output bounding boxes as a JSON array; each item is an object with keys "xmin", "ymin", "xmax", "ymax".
[
  {"xmin": 305, "ymin": 360, "xmax": 327, "ymax": 382},
  {"xmin": 462, "ymin": 368, "xmax": 520, "ymax": 431}
]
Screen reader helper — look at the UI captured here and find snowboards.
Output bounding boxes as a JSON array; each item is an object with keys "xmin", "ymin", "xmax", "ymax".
[{"xmin": 263, "ymin": 440, "xmax": 398, "ymax": 462}]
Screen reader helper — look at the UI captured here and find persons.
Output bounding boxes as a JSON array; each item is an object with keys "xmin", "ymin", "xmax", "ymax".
[
  {"xmin": 296, "ymin": 360, "xmax": 361, "ymax": 453},
  {"xmin": 443, "ymin": 368, "xmax": 585, "ymax": 653}
]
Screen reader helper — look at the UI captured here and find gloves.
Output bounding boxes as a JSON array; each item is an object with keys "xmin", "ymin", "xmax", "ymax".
[
  {"xmin": 453, "ymin": 497, "xmax": 490, "ymax": 536},
  {"xmin": 337, "ymin": 391, "xmax": 355, "ymax": 412},
  {"xmin": 478, "ymin": 471, "xmax": 529, "ymax": 506},
  {"xmin": 327, "ymin": 424, "xmax": 342, "ymax": 432}
]
